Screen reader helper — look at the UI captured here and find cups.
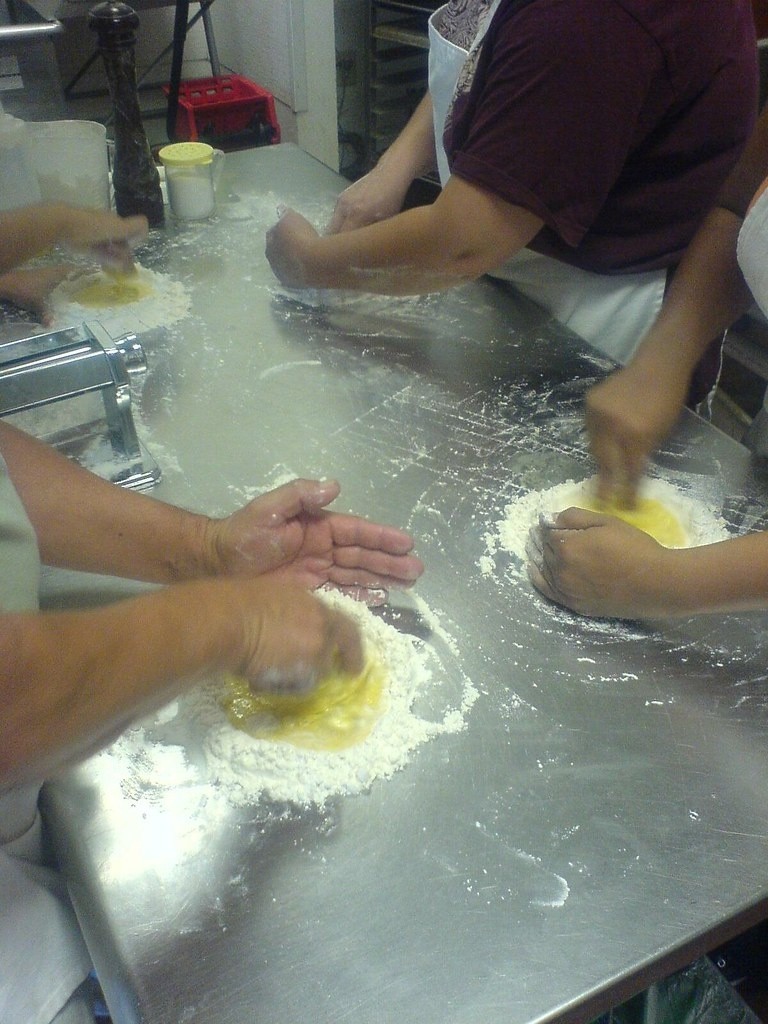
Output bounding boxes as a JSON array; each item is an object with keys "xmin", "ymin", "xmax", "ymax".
[{"xmin": 159, "ymin": 141, "xmax": 225, "ymax": 219}]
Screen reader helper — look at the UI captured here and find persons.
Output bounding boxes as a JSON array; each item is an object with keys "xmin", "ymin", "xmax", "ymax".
[
  {"xmin": 0, "ymin": 203, "xmax": 148, "ymax": 328},
  {"xmin": 0, "ymin": 423, "xmax": 424, "ymax": 1023},
  {"xmin": 265, "ymin": 1, "xmax": 768, "ymax": 420},
  {"xmin": 528, "ymin": 110, "xmax": 768, "ymax": 620}
]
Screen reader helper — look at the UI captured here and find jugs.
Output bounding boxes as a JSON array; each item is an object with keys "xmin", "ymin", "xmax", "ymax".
[{"xmin": 19, "ymin": 120, "xmax": 115, "ymax": 213}]
